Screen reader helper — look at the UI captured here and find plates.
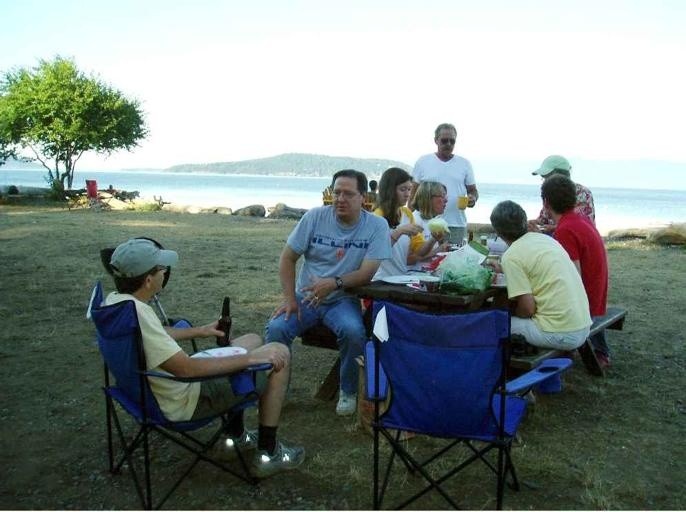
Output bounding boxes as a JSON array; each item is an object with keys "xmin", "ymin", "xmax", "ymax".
[
  {"xmin": 189, "ymin": 347, "xmax": 248, "ymax": 359},
  {"xmin": 489, "ymin": 273, "xmax": 507, "ymax": 287},
  {"xmin": 382, "ymin": 275, "xmax": 440, "ymax": 284}
]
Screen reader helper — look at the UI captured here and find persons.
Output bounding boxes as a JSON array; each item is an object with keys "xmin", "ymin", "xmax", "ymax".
[
  {"xmin": 366, "ymin": 180, "xmax": 380, "ymax": 194},
  {"xmin": 262, "ymin": 169, "xmax": 395, "ymax": 417},
  {"xmin": 409, "ymin": 179, "xmax": 456, "ymax": 261},
  {"xmin": 363, "ymin": 166, "xmax": 450, "ymax": 310},
  {"xmin": 100, "ymin": 238, "xmax": 307, "ymax": 482},
  {"xmin": 486, "ymin": 199, "xmax": 594, "ymax": 411},
  {"xmin": 525, "ymin": 154, "xmax": 613, "ymax": 373},
  {"xmin": 541, "ymin": 173, "xmax": 609, "ymax": 316},
  {"xmin": 106, "ymin": 184, "xmax": 115, "ymax": 191},
  {"xmin": 406, "ymin": 122, "xmax": 479, "ymax": 246}
]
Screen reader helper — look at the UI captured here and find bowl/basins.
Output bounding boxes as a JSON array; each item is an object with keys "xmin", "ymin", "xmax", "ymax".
[
  {"xmin": 487, "ymin": 238, "xmax": 507, "ymax": 251},
  {"xmin": 420, "ymin": 276, "xmax": 440, "ymax": 291}
]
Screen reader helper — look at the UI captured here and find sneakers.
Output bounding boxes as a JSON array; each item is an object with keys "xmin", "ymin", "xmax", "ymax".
[
  {"xmin": 218, "ymin": 427, "xmax": 258, "ymax": 460},
  {"xmin": 336, "ymin": 390, "xmax": 357, "ymax": 416},
  {"xmin": 249, "ymin": 441, "xmax": 304, "ymax": 478}
]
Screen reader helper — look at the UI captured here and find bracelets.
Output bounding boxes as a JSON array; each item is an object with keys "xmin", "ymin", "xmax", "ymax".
[{"xmin": 391, "ymin": 235, "xmax": 398, "ymax": 242}]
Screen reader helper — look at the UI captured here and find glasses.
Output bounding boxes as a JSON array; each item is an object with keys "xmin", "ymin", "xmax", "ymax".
[
  {"xmin": 157, "ymin": 266, "xmax": 167, "ymax": 273},
  {"xmin": 431, "ymin": 194, "xmax": 447, "ymax": 199},
  {"xmin": 440, "ymin": 138, "xmax": 455, "ymax": 144},
  {"xmin": 332, "ymin": 190, "xmax": 355, "ymax": 198}
]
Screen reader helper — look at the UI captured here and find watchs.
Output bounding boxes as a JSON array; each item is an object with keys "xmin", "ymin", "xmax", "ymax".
[{"xmin": 333, "ymin": 275, "xmax": 343, "ymax": 291}]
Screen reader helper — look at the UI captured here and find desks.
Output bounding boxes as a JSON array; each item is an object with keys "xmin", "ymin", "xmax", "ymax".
[{"xmin": 300, "ymin": 251, "xmax": 629, "ymax": 401}]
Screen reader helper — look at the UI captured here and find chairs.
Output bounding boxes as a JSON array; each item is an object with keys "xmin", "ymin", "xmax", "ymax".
[
  {"xmin": 79, "ymin": 282, "xmax": 274, "ymax": 507},
  {"xmin": 360, "ymin": 297, "xmax": 574, "ymax": 507}
]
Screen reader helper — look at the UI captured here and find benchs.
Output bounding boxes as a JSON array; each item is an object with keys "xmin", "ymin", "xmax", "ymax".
[
  {"xmin": 300, "ymin": 314, "xmax": 360, "ymax": 405},
  {"xmin": 498, "ymin": 308, "xmax": 632, "ymax": 401}
]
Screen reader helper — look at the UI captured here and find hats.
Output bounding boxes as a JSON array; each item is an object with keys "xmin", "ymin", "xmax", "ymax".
[
  {"xmin": 108, "ymin": 239, "xmax": 178, "ymax": 278},
  {"xmin": 531, "ymin": 155, "xmax": 570, "ymax": 176}
]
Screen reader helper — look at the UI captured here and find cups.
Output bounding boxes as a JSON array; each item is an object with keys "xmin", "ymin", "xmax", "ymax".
[{"xmin": 456, "ymin": 193, "xmax": 470, "ymax": 210}]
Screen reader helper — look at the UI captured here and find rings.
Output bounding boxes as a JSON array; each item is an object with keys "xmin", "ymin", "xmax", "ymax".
[{"xmin": 314, "ymin": 296, "xmax": 319, "ymax": 300}]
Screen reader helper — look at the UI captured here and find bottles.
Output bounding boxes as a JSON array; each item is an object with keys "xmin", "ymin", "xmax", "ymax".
[{"xmin": 215, "ymin": 296, "xmax": 232, "ymax": 345}]
